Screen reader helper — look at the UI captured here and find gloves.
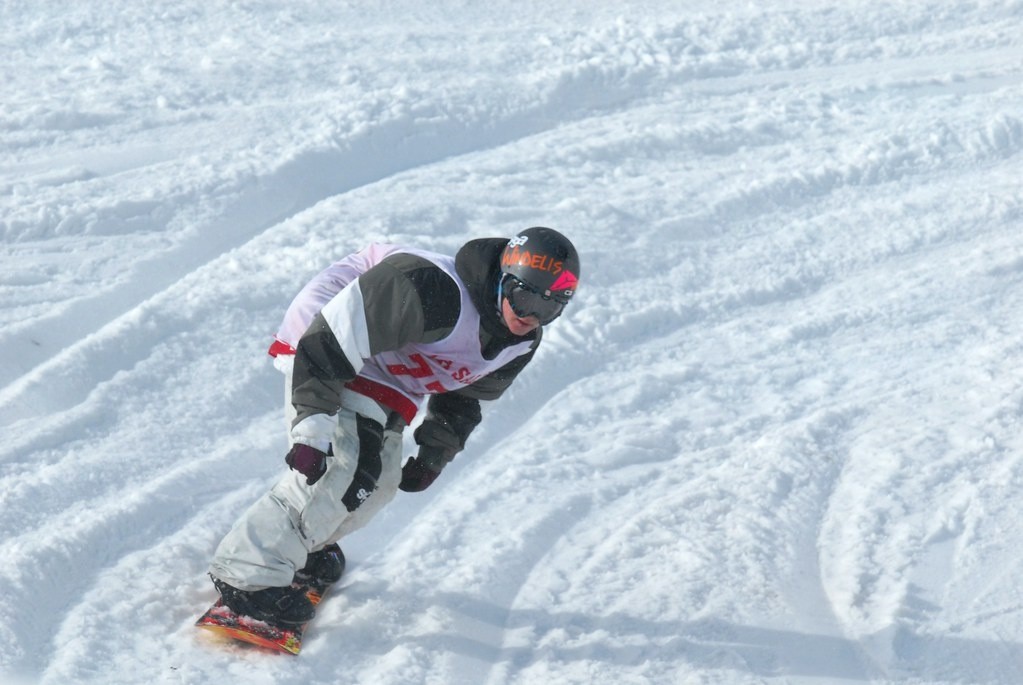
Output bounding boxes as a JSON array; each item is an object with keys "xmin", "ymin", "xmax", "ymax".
[
  {"xmin": 285, "ymin": 443, "xmax": 327, "ymax": 485},
  {"xmin": 398, "ymin": 456, "xmax": 441, "ymax": 492}
]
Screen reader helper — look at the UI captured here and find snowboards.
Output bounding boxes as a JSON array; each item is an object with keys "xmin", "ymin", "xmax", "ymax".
[{"xmin": 194, "ymin": 538, "xmax": 347, "ymax": 657}]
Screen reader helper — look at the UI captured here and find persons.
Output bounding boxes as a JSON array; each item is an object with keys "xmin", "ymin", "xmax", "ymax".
[{"xmin": 199, "ymin": 227, "xmax": 579, "ymax": 625}]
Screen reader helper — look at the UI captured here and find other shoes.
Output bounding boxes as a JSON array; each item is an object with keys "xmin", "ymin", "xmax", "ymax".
[
  {"xmin": 297, "ymin": 543, "xmax": 345, "ymax": 586},
  {"xmin": 207, "ymin": 572, "xmax": 316, "ymax": 624}
]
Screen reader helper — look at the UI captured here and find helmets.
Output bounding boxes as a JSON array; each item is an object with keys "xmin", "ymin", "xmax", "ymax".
[{"xmin": 499, "ymin": 227, "xmax": 579, "ymax": 304}]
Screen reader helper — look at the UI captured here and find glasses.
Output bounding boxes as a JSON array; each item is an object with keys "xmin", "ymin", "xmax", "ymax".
[{"xmin": 500, "ymin": 273, "xmax": 568, "ymax": 325}]
